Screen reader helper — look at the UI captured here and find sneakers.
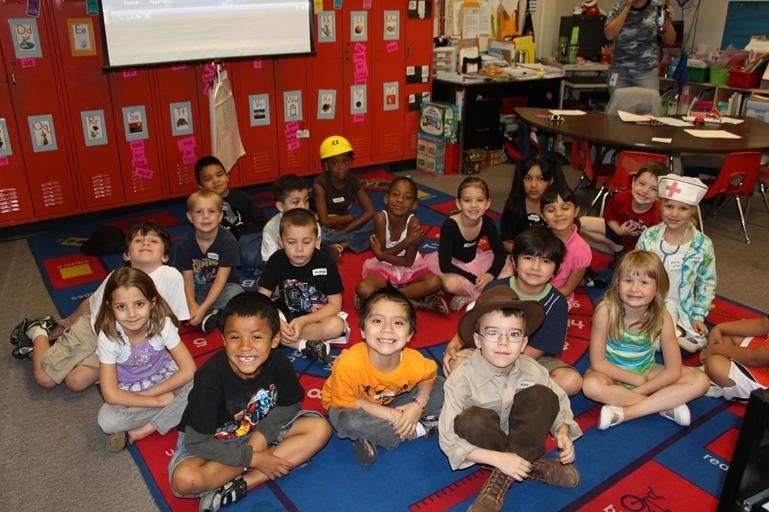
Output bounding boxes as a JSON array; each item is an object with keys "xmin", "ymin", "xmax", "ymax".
[
  {"xmin": 450, "ymin": 295, "xmax": 476, "ymax": 312},
  {"xmin": 468, "ymin": 466, "xmax": 515, "ymax": 512},
  {"xmin": 658, "ymin": 403, "xmax": 693, "ymax": 428},
  {"xmin": 597, "ymin": 404, "xmax": 625, "ymax": 430},
  {"xmin": 10, "ymin": 314, "xmax": 59, "ymax": 362},
  {"xmin": 526, "ymin": 456, "xmax": 580, "ymax": 489},
  {"xmin": 199, "ymin": 474, "xmax": 248, "ymax": 512},
  {"xmin": 416, "ymin": 293, "xmax": 450, "ymax": 317},
  {"xmin": 301, "ymin": 339, "xmax": 331, "ymax": 365},
  {"xmin": 353, "ymin": 437, "xmax": 378, "ymax": 467},
  {"xmin": 107, "ymin": 429, "xmax": 134, "ymax": 451},
  {"xmin": 419, "ymin": 408, "xmax": 441, "ymax": 439},
  {"xmin": 200, "ymin": 308, "xmax": 223, "ymax": 335}
]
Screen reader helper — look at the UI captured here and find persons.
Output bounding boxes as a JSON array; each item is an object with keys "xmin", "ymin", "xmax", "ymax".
[
  {"xmin": 603, "ymin": 0, "xmax": 676, "ymax": 97},
  {"xmin": 8, "ymin": 221, "xmax": 190, "ymax": 391},
  {"xmin": 167, "ymin": 290, "xmax": 334, "ymax": 510},
  {"xmin": 320, "ymin": 286, "xmax": 447, "ymax": 466},
  {"xmin": 94, "ymin": 266, "xmax": 197, "ymax": 452},
  {"xmin": 697, "ymin": 317, "xmax": 769, "ymax": 407},
  {"xmin": 636, "ymin": 173, "xmax": 718, "ymax": 360},
  {"xmin": 438, "ymin": 285, "xmax": 584, "ymax": 512},
  {"xmin": 581, "ymin": 249, "xmax": 711, "ymax": 431}
]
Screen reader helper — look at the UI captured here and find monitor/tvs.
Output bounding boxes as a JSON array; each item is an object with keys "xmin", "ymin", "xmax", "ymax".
[{"xmin": 569, "ymin": 14, "xmax": 615, "ymax": 61}]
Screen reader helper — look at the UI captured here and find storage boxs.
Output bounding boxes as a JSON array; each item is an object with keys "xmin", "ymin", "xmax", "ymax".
[
  {"xmin": 729, "ymin": 69, "xmax": 761, "ymax": 88},
  {"xmin": 686, "ymin": 63, "xmax": 710, "ymax": 82},
  {"xmin": 710, "ymin": 68, "xmax": 728, "ymax": 84}
]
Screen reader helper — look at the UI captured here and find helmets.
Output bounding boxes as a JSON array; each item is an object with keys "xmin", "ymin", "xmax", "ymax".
[{"xmin": 319, "ymin": 135, "xmax": 354, "ymax": 160}]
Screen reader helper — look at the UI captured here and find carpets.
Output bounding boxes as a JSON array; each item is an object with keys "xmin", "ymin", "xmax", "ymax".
[{"xmin": 26, "ymin": 166, "xmax": 768, "ymax": 511}]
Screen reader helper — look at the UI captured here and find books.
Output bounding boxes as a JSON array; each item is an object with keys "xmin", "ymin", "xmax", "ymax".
[{"xmin": 727, "ymin": 92, "xmax": 749, "ymax": 117}]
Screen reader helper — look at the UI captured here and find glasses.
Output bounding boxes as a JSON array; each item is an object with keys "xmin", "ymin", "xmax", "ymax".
[{"xmin": 475, "ymin": 330, "xmax": 525, "ymax": 343}]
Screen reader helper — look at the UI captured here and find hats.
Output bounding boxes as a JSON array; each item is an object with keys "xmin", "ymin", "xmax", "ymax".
[
  {"xmin": 458, "ymin": 285, "xmax": 545, "ymax": 348},
  {"xmin": 657, "ymin": 173, "xmax": 709, "ymax": 206}
]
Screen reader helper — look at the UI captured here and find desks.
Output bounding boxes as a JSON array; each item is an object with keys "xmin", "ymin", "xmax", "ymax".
[
  {"xmin": 540, "ymin": 56, "xmax": 610, "ymax": 108},
  {"xmin": 513, "ymin": 108, "xmax": 769, "ymax": 235},
  {"xmin": 657, "ymin": 77, "xmax": 769, "ymax": 124},
  {"xmin": 434, "ymin": 72, "xmax": 563, "ymax": 174}
]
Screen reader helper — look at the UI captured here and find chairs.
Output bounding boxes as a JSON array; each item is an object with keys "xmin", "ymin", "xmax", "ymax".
[
  {"xmin": 598, "ymin": 148, "xmax": 669, "ymax": 220},
  {"xmin": 582, "ymin": 143, "xmax": 612, "ymax": 204},
  {"xmin": 569, "ymin": 136, "xmax": 590, "ymax": 197},
  {"xmin": 605, "ymin": 87, "xmax": 664, "ymax": 115},
  {"xmin": 694, "ymin": 150, "xmax": 762, "ymax": 246}
]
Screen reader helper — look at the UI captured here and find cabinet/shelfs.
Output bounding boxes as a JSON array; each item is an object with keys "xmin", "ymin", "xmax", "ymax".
[{"xmin": 0, "ymin": 0, "xmax": 438, "ymax": 243}]
